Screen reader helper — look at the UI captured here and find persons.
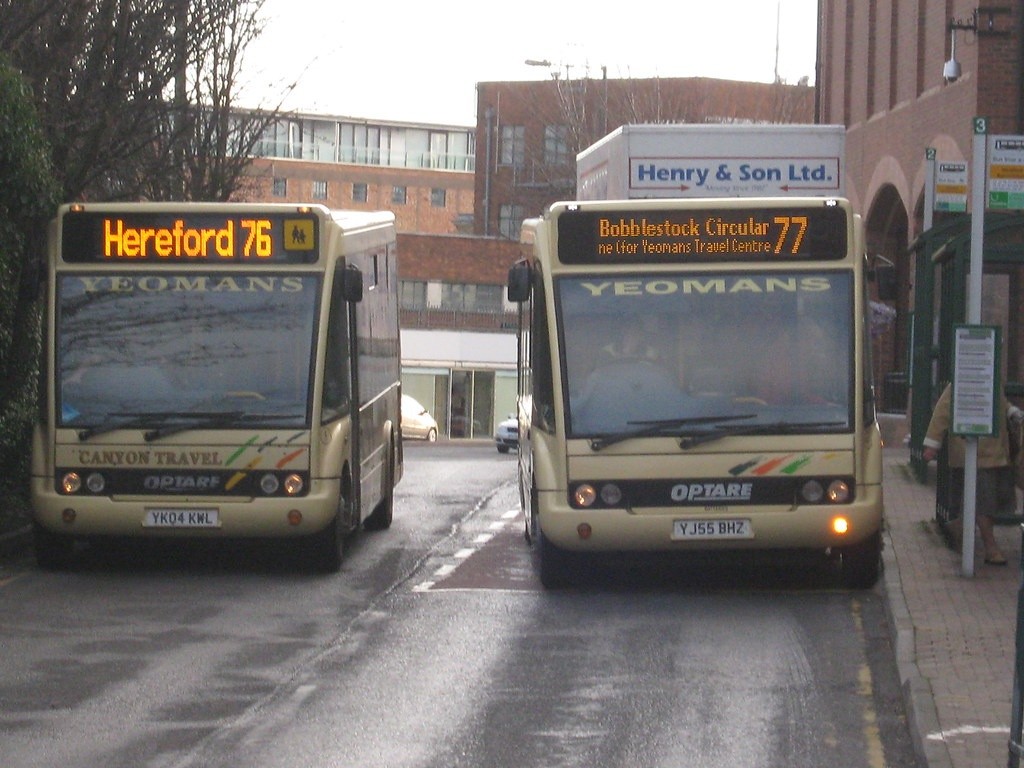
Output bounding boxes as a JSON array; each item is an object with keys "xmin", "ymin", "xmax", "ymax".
[
  {"xmin": 590, "ymin": 311, "xmax": 670, "ymax": 380},
  {"xmin": 921, "ymin": 372, "xmax": 1024, "ymax": 565},
  {"xmin": 62, "ymin": 323, "xmax": 152, "ymax": 394}
]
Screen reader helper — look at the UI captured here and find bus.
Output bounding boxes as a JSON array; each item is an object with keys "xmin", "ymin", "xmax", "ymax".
[
  {"xmin": 31, "ymin": 201, "xmax": 403, "ymax": 573},
  {"xmin": 507, "ymin": 195, "xmax": 886, "ymax": 560}
]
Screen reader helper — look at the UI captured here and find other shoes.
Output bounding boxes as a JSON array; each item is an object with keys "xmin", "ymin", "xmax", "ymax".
[
  {"xmin": 984, "ymin": 546, "xmax": 1007, "ymax": 565},
  {"xmin": 944, "ymin": 517, "xmax": 962, "ymax": 553}
]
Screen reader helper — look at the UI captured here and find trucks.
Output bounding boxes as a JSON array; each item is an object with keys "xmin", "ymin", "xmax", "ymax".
[{"xmin": 576, "ymin": 123, "xmax": 847, "ymax": 198}]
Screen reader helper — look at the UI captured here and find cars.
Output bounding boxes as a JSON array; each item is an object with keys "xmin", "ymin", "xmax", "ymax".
[
  {"xmin": 494, "ymin": 412, "xmax": 519, "ymax": 453},
  {"xmin": 401, "ymin": 393, "xmax": 439, "ymax": 442}
]
